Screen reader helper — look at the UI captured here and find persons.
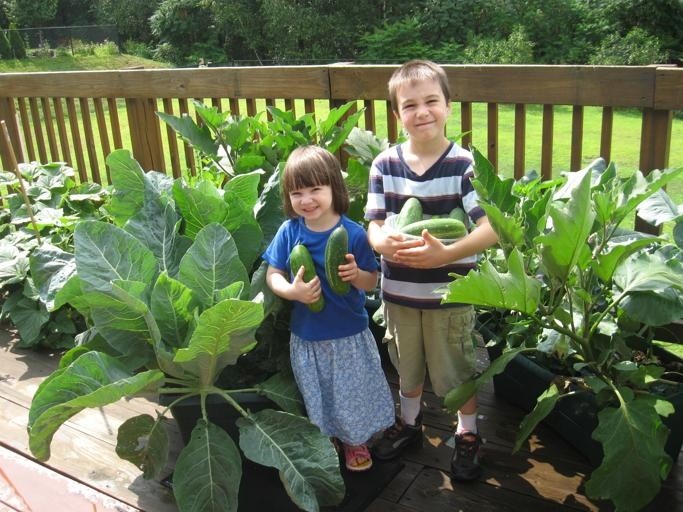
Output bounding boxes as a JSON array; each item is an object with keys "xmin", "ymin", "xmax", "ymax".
[
  {"xmin": 260, "ymin": 145, "xmax": 394, "ymax": 473},
  {"xmin": 360, "ymin": 58, "xmax": 498, "ymax": 484}
]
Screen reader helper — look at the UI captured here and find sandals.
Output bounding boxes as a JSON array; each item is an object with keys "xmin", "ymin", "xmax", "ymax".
[{"xmin": 333, "ymin": 437, "xmax": 372, "ymax": 470}]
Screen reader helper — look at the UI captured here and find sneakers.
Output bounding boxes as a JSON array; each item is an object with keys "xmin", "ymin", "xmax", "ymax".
[
  {"xmin": 374, "ymin": 414, "xmax": 422, "ymax": 459},
  {"xmin": 449, "ymin": 430, "xmax": 480, "ymax": 481}
]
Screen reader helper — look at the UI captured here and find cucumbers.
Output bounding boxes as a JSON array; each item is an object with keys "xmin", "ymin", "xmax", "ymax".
[
  {"xmin": 325, "ymin": 224, "xmax": 352, "ymax": 296},
  {"xmin": 396, "ymin": 197, "xmax": 467, "ymax": 244},
  {"xmin": 290, "ymin": 244, "xmax": 326, "ymax": 313}
]
